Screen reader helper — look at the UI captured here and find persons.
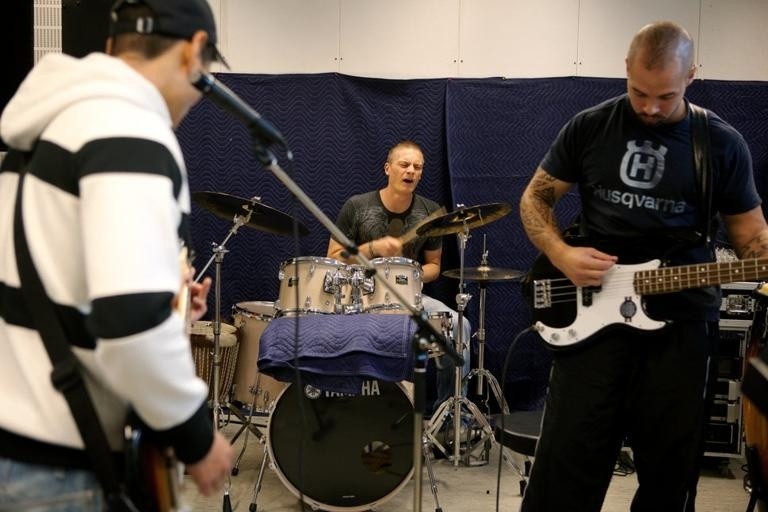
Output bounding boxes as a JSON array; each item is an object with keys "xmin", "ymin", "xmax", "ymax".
[
  {"xmin": 518, "ymin": 21, "xmax": 768, "ymax": 512},
  {"xmin": 0, "ymin": 1, "xmax": 231, "ymax": 511},
  {"xmin": 326, "ymin": 140, "xmax": 471, "ymax": 415}
]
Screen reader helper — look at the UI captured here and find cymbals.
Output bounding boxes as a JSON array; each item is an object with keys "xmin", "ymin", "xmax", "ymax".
[
  {"xmin": 443, "ymin": 266, "xmax": 524, "ymax": 280},
  {"xmin": 417, "ymin": 203, "xmax": 513, "ymax": 237},
  {"xmin": 190, "ymin": 190, "xmax": 310, "ymax": 238}
]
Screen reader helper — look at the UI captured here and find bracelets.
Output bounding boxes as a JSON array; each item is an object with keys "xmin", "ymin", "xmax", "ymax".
[{"xmin": 368, "ymin": 241, "xmax": 376, "ymax": 258}]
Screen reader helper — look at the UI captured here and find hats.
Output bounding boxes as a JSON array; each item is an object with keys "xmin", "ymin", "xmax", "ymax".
[{"xmin": 103, "ymin": 0, "xmax": 233, "ymax": 74}]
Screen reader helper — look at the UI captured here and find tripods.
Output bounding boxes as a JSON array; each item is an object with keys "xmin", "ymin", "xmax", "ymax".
[
  {"xmin": 453, "ymin": 285, "xmax": 532, "ymax": 476},
  {"xmin": 421, "ymin": 230, "xmax": 528, "ymax": 497}
]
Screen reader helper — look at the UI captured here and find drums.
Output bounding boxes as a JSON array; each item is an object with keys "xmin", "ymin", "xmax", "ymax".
[
  {"xmin": 191, "ymin": 320, "xmax": 240, "ymax": 404},
  {"xmin": 274, "ymin": 256, "xmax": 356, "ymax": 317},
  {"xmin": 352, "ymin": 257, "xmax": 422, "ymax": 315},
  {"xmin": 427, "ymin": 310, "xmax": 454, "ymax": 359},
  {"xmin": 231, "ymin": 301, "xmax": 291, "ymax": 415},
  {"xmin": 268, "ymin": 373, "xmax": 422, "ymax": 512}
]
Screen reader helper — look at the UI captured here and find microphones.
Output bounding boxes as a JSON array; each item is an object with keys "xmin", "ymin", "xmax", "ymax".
[{"xmin": 191, "ymin": 69, "xmax": 286, "ymax": 146}]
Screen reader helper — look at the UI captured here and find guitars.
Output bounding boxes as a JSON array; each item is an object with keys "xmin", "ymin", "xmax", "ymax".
[
  {"xmin": 122, "ymin": 236, "xmax": 197, "ymax": 511},
  {"xmin": 524, "ymin": 247, "xmax": 768, "ymax": 351}
]
